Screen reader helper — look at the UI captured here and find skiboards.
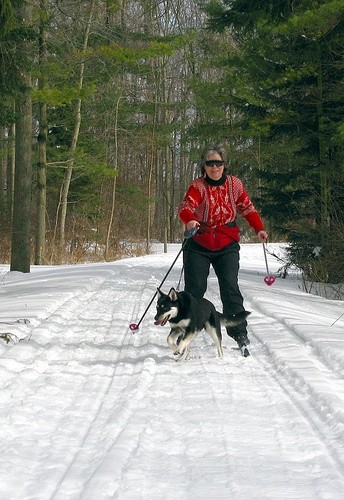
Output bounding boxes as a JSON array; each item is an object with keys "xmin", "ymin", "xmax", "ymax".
[{"xmin": 240, "ymin": 345, "xmax": 250, "ymax": 357}]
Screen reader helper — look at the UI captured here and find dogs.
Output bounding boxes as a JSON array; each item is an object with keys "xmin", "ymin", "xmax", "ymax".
[{"xmin": 154, "ymin": 287, "xmax": 252, "ymax": 364}]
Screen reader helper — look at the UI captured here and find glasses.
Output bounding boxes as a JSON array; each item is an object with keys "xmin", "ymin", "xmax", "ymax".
[{"xmin": 204, "ymin": 159, "xmax": 222, "ymax": 167}]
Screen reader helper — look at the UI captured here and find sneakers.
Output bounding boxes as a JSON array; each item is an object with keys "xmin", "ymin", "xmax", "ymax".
[{"xmin": 234, "ymin": 335, "xmax": 250, "ymax": 346}]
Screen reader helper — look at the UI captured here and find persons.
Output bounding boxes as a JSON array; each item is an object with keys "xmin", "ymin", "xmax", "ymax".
[{"xmin": 177, "ymin": 144, "xmax": 268, "ymax": 346}]
